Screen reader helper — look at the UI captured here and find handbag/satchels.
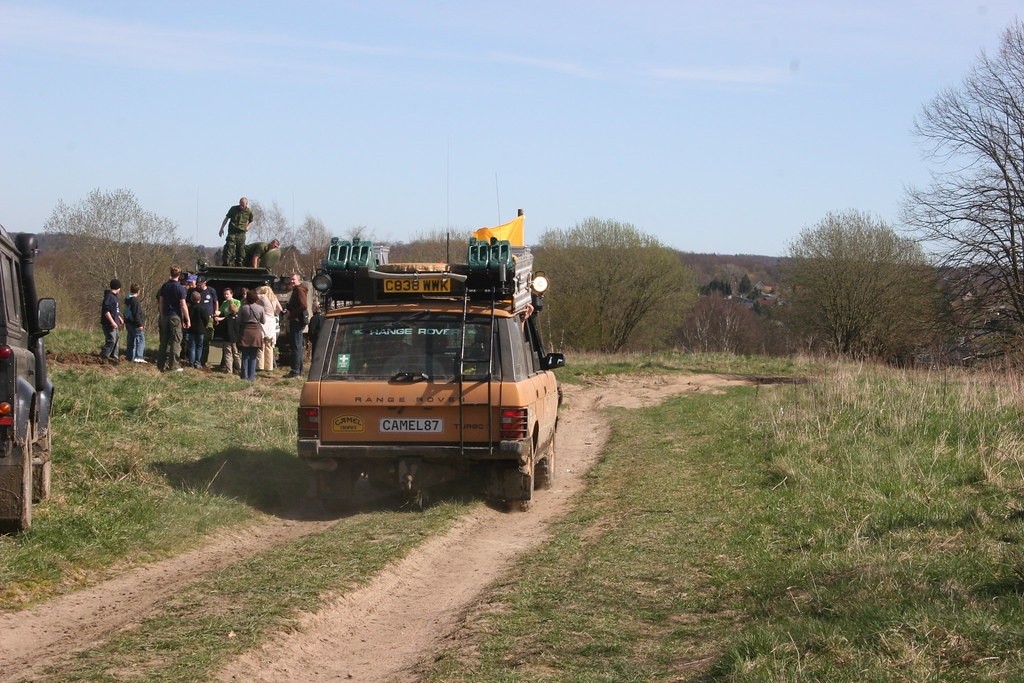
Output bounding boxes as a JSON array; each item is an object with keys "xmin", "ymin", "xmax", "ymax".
[{"xmin": 300, "ymin": 309, "xmax": 308, "ymax": 325}]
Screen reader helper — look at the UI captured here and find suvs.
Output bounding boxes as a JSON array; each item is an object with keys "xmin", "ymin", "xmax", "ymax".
[
  {"xmin": 298, "ymin": 235, "xmax": 567, "ymax": 511},
  {"xmin": 0, "ymin": 225, "xmax": 55, "ymax": 532}
]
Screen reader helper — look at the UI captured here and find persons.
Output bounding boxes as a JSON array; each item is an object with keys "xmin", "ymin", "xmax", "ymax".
[
  {"xmin": 99, "ymin": 279, "xmax": 124, "ymax": 361},
  {"xmin": 283, "ymin": 272, "xmax": 309, "ymax": 378},
  {"xmin": 520, "ymin": 303, "xmax": 535, "ymax": 333},
  {"xmin": 244, "ymin": 240, "xmax": 280, "ymax": 268},
  {"xmin": 219, "ymin": 197, "xmax": 254, "ymax": 266},
  {"xmin": 157, "ymin": 266, "xmax": 283, "ymax": 382},
  {"xmin": 125, "ymin": 284, "xmax": 148, "ymax": 364}
]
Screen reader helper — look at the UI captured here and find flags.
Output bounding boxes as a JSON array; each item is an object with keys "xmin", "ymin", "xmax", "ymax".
[{"xmin": 472, "ymin": 215, "xmax": 524, "ymax": 247}]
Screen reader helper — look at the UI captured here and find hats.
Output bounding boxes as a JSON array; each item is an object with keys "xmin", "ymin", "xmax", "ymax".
[
  {"xmin": 196, "ymin": 276, "xmax": 205, "ymax": 285},
  {"xmin": 186, "ymin": 274, "xmax": 197, "ymax": 282},
  {"xmin": 110, "ymin": 279, "xmax": 121, "ymax": 289}
]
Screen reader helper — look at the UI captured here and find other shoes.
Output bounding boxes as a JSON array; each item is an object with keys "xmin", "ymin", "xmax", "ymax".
[
  {"xmin": 111, "ymin": 355, "xmax": 119, "ymax": 360},
  {"xmin": 222, "ymin": 370, "xmax": 233, "ymax": 374},
  {"xmin": 195, "ymin": 363, "xmax": 203, "ymax": 369},
  {"xmin": 283, "ymin": 371, "xmax": 300, "ymax": 379},
  {"xmin": 159, "ymin": 367, "xmax": 166, "ymax": 372},
  {"xmin": 176, "ymin": 368, "xmax": 183, "ymax": 372},
  {"xmin": 133, "ymin": 358, "xmax": 147, "ymax": 364},
  {"xmin": 189, "ymin": 365, "xmax": 194, "ymax": 369},
  {"xmin": 98, "ymin": 354, "xmax": 108, "ymax": 364},
  {"xmin": 234, "ymin": 369, "xmax": 241, "ymax": 374}
]
